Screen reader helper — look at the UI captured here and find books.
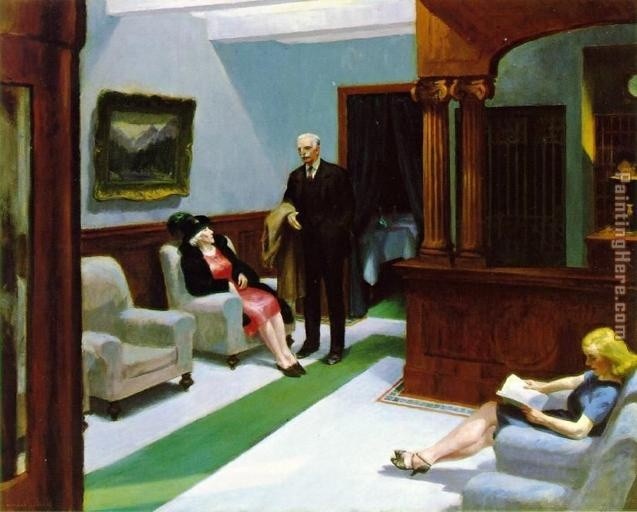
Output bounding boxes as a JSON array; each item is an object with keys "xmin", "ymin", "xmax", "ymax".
[{"xmin": 494, "ymin": 373, "xmax": 550, "ymax": 415}]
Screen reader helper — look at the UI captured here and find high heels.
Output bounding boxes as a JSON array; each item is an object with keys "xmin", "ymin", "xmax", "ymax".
[{"xmin": 391, "ymin": 449, "xmax": 431, "ymax": 477}]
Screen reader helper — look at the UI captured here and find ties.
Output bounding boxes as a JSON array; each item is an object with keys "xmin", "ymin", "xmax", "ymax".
[{"xmin": 306, "ymin": 165, "xmax": 314, "ymax": 183}]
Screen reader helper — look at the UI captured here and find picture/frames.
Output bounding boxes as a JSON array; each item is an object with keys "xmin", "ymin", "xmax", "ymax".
[{"xmin": 92, "ymin": 89, "xmax": 197, "ymax": 204}]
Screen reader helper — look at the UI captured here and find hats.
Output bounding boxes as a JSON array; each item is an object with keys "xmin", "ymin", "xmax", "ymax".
[{"xmin": 177, "ymin": 212, "xmax": 211, "ymax": 242}]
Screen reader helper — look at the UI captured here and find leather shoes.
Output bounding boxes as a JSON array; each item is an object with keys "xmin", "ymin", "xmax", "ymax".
[
  {"xmin": 294, "ymin": 340, "xmax": 319, "ymax": 358},
  {"xmin": 277, "ymin": 360, "xmax": 305, "ymax": 378},
  {"xmin": 324, "ymin": 346, "xmax": 344, "ymax": 365}
]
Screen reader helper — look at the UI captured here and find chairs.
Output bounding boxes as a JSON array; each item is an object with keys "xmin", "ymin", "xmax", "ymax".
[
  {"xmin": 80, "ymin": 236, "xmax": 295, "ymax": 420},
  {"xmin": 464, "ymin": 362, "xmax": 636, "ymax": 512},
  {"xmin": 363, "ymin": 205, "xmax": 417, "ymax": 310}
]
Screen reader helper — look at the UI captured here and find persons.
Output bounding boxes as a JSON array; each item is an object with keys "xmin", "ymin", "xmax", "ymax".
[
  {"xmin": 278, "ymin": 133, "xmax": 357, "ymax": 366},
  {"xmin": 178, "ymin": 214, "xmax": 307, "ymax": 379},
  {"xmin": 386, "ymin": 324, "xmax": 636, "ymax": 479}
]
